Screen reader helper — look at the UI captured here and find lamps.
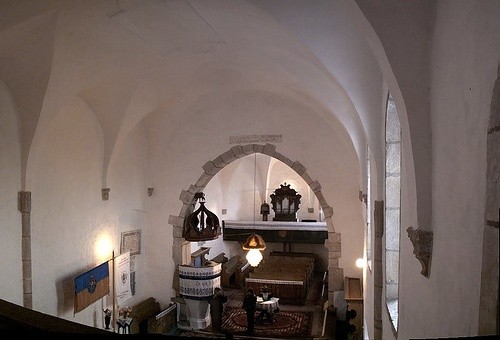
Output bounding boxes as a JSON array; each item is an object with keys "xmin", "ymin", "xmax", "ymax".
[{"xmin": 243, "ymin": 152, "xmax": 267, "ymax": 269}]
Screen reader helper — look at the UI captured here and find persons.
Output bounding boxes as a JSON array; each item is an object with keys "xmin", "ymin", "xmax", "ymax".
[
  {"xmin": 242, "ymin": 288, "xmax": 256, "ymax": 335},
  {"xmin": 207, "ymin": 288, "xmax": 228, "ymax": 332}
]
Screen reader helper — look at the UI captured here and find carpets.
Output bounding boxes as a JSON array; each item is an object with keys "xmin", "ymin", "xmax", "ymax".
[{"xmin": 217, "ymin": 305, "xmax": 313, "ymax": 340}]
